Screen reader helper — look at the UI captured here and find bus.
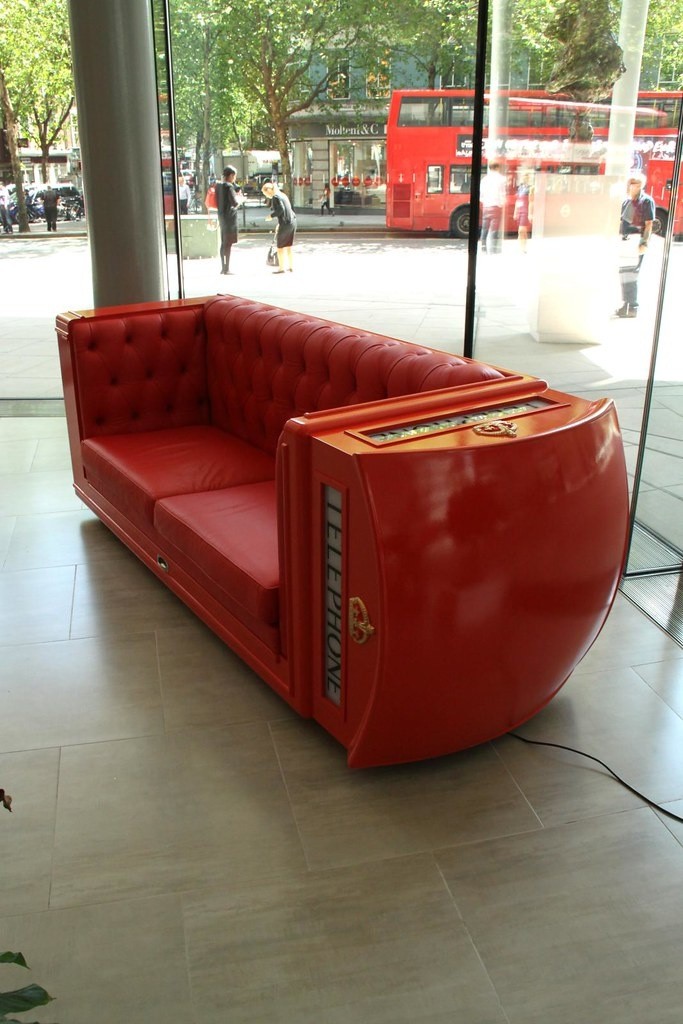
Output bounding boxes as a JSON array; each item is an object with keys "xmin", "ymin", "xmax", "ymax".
[{"xmin": 384, "ymin": 89, "xmax": 683, "ymax": 242}]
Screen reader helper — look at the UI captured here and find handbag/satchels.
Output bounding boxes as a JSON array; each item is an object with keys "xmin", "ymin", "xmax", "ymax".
[
  {"xmin": 267, "ymin": 232, "xmax": 279, "ymax": 266},
  {"xmin": 205, "ymin": 182, "xmax": 218, "ymax": 209}
]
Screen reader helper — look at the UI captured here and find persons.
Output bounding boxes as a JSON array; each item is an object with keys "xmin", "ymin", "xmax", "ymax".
[
  {"xmin": 0, "ymin": 181, "xmax": 13, "ymax": 234},
  {"xmin": 261, "ymin": 183, "xmax": 297, "ymax": 274},
  {"xmin": 615, "ymin": 174, "xmax": 655, "ymax": 319},
  {"xmin": 43, "ymin": 186, "xmax": 57, "ymax": 232},
  {"xmin": 513, "ymin": 172, "xmax": 534, "ymax": 248},
  {"xmin": 318, "ymin": 183, "xmax": 336, "ymax": 217},
  {"xmin": 215, "ymin": 166, "xmax": 240, "ymax": 275},
  {"xmin": 178, "ymin": 177, "xmax": 191, "ymax": 215},
  {"xmin": 480, "ymin": 160, "xmax": 507, "ymax": 255},
  {"xmin": 24, "ymin": 189, "xmax": 31, "ymax": 207}
]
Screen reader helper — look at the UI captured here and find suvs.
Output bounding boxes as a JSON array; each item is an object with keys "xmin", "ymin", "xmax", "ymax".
[{"xmin": 28, "ymin": 183, "xmax": 85, "ymax": 216}]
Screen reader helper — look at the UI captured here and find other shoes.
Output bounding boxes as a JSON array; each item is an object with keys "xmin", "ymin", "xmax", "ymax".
[
  {"xmin": 273, "ymin": 269, "xmax": 292, "ymax": 274},
  {"xmin": 220, "ymin": 271, "xmax": 233, "ymax": 275},
  {"xmin": 616, "ymin": 304, "xmax": 637, "ymax": 317}
]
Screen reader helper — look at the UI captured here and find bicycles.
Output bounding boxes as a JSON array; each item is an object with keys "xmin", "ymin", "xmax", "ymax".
[{"xmin": 0, "ymin": 195, "xmax": 13, "ymax": 233}]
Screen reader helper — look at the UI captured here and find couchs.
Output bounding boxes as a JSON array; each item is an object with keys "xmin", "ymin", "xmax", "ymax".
[{"xmin": 55, "ymin": 294, "xmax": 629, "ymax": 770}]
